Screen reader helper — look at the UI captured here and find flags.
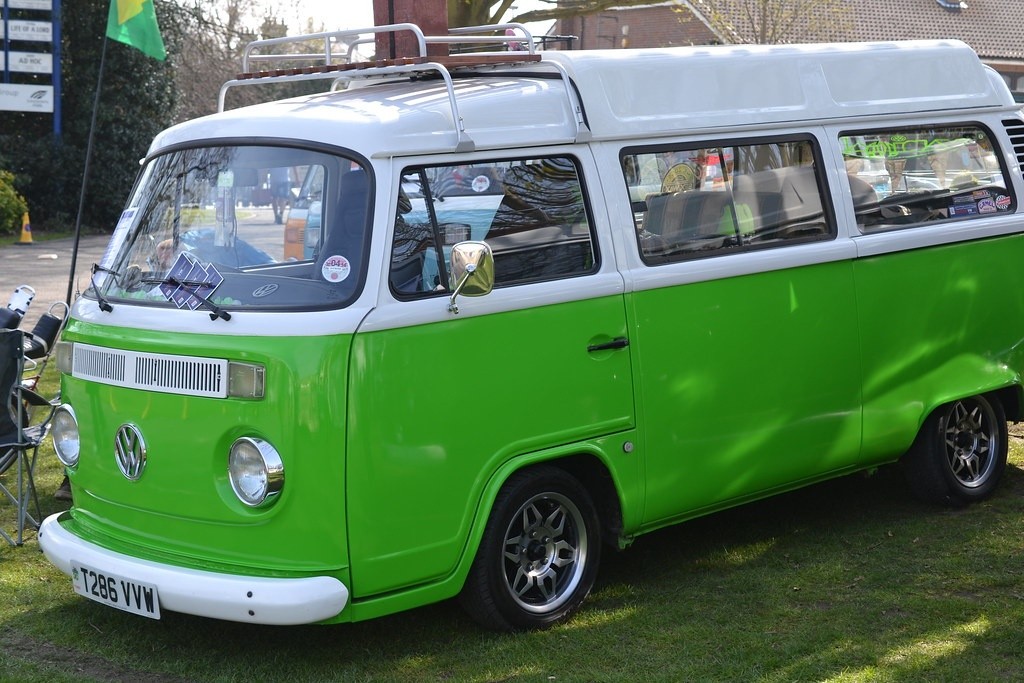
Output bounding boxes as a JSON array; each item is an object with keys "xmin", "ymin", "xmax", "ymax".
[{"xmin": 106, "ymin": 0, "xmax": 168, "ymax": 62}]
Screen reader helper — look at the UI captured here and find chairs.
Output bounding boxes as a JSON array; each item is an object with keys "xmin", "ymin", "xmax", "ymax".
[
  {"xmin": 484, "ymin": 165, "xmax": 588, "ymax": 282},
  {"xmin": 310, "ymin": 170, "xmax": 429, "ymax": 292},
  {"xmin": 777, "ymin": 169, "xmax": 877, "ymax": 238},
  {"xmin": 0, "ymin": 328, "xmax": 61, "ymax": 547}
]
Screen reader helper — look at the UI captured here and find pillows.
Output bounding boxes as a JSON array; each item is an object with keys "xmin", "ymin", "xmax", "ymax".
[{"xmin": 641, "ymin": 190, "xmax": 727, "ymax": 239}]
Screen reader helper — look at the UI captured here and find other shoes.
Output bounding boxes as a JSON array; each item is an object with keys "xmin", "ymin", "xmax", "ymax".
[
  {"xmin": 54, "ymin": 477, "xmax": 73, "ymax": 501},
  {"xmin": 276, "ymin": 212, "xmax": 283, "ymax": 226}
]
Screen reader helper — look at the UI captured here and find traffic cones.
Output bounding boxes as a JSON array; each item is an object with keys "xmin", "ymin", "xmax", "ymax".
[{"xmin": 14, "ymin": 212, "xmax": 37, "ymax": 246}]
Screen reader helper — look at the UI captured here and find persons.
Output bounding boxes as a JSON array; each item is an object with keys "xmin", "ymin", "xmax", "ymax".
[
  {"xmin": 270, "ymin": 165, "xmax": 289, "ymax": 224},
  {"xmin": 156, "ymin": 228, "xmax": 273, "ymax": 271}
]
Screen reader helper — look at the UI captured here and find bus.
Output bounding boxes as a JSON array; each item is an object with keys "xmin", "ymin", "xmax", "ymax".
[{"xmin": 39, "ymin": 21, "xmax": 1024, "ymax": 634}]
